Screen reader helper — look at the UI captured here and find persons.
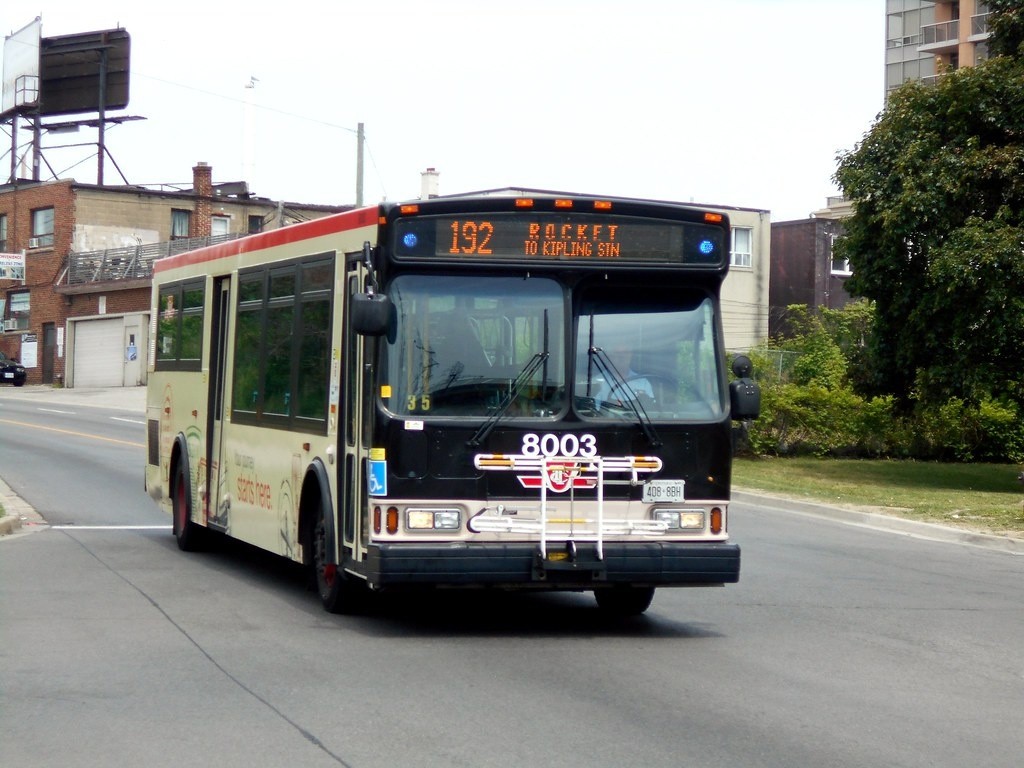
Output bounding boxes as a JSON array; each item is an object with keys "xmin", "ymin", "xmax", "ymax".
[{"xmin": 591, "ymin": 338, "xmax": 655, "ymax": 411}]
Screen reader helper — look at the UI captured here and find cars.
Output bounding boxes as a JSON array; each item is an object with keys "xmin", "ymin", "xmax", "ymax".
[{"xmin": 0, "ymin": 352, "xmax": 27, "ymax": 387}]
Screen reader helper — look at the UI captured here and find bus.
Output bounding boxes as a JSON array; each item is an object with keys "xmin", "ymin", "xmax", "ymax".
[{"xmin": 144, "ymin": 186, "xmax": 763, "ymax": 617}]
